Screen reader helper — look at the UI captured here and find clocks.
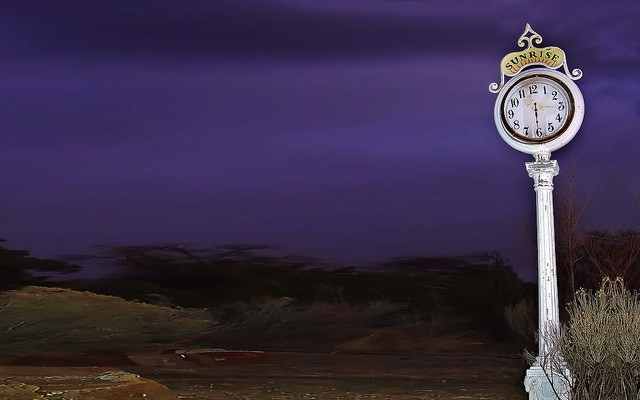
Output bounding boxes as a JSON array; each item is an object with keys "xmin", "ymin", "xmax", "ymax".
[{"xmin": 489, "ymin": 23, "xmax": 585, "ymax": 154}]
